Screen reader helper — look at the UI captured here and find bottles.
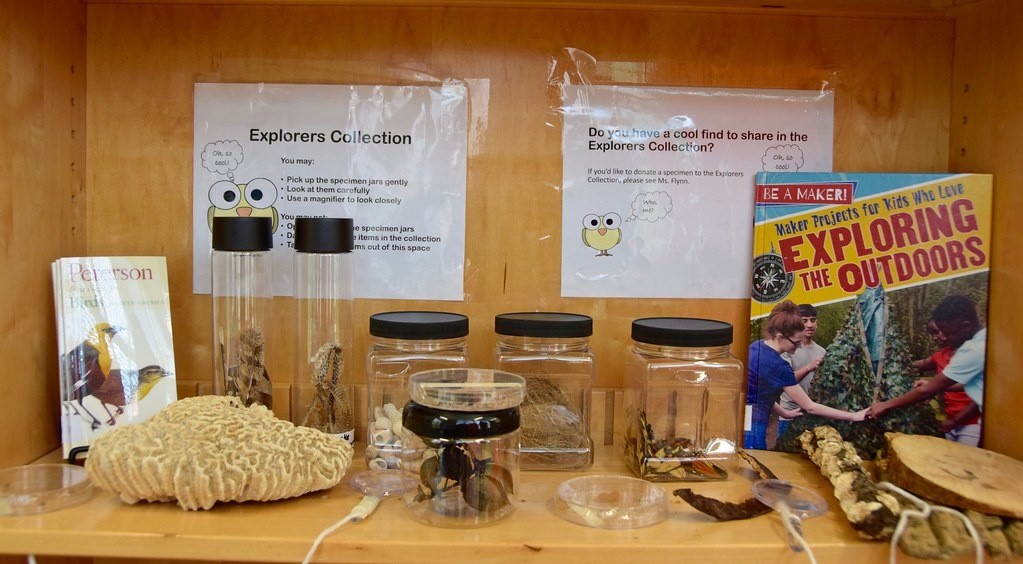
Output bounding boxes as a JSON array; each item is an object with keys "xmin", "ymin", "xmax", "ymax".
[
  {"xmin": 493, "ymin": 310, "xmax": 596, "ymax": 471},
  {"xmin": 366, "ymin": 309, "xmax": 470, "ymax": 476},
  {"xmin": 291, "ymin": 216, "xmax": 360, "ymax": 447},
  {"xmin": 623, "ymin": 316, "xmax": 744, "ymax": 487},
  {"xmin": 399, "ymin": 398, "xmax": 526, "ymax": 529},
  {"xmin": 208, "ymin": 216, "xmax": 290, "ymax": 412}
]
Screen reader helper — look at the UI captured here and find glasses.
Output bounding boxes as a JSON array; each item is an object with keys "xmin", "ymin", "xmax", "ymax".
[{"xmin": 780, "ymin": 329, "xmax": 801, "ymax": 348}]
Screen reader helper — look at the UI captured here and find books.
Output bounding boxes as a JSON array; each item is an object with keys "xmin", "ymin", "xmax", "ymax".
[
  {"xmin": 741, "ymin": 170, "xmax": 995, "ymax": 461},
  {"xmin": 52, "ymin": 256, "xmax": 179, "ymax": 461}
]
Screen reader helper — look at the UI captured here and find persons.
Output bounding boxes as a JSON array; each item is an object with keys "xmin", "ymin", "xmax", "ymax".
[
  {"xmin": 742, "ymin": 300, "xmax": 876, "ymax": 450},
  {"xmin": 910, "ymin": 316, "xmax": 982, "ymax": 448},
  {"xmin": 865, "ymin": 294, "xmax": 987, "ymax": 420},
  {"xmin": 778, "ymin": 303, "xmax": 827, "ymax": 442}
]
